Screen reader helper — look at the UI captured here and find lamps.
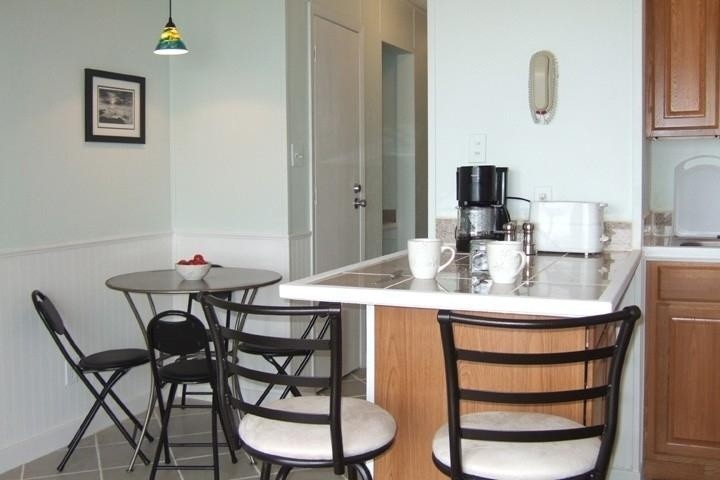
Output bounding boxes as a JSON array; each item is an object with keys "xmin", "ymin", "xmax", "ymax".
[{"xmin": 146, "ymin": 0, "xmax": 190, "ymax": 56}]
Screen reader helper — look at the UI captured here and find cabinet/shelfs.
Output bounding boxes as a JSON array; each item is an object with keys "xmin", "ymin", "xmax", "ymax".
[
  {"xmin": 643, "ymin": 0, "xmax": 720, "ymax": 138},
  {"xmin": 644, "ymin": 260, "xmax": 720, "ymax": 479}
]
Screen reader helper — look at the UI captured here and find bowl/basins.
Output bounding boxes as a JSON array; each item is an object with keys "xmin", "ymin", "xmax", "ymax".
[
  {"xmin": 174, "ymin": 263, "xmax": 212, "ymax": 281},
  {"xmin": 175, "ymin": 279, "xmax": 211, "ymax": 290}
]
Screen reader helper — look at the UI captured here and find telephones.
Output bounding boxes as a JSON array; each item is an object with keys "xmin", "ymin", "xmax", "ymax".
[{"xmin": 529, "ymin": 50, "xmax": 558, "ymax": 124}]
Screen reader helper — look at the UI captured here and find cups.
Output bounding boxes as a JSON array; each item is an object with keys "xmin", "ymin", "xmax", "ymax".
[
  {"xmin": 650, "ymin": 210, "xmax": 671, "ymax": 236},
  {"xmin": 469, "ymin": 239, "xmax": 493, "ymax": 270},
  {"xmin": 489, "ymin": 240, "xmax": 525, "ymax": 283},
  {"xmin": 406, "ymin": 238, "xmax": 457, "ymax": 278}
]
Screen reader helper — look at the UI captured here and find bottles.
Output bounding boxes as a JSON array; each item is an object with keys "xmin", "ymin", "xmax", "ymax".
[
  {"xmin": 521, "ymin": 222, "xmax": 537, "ymax": 255},
  {"xmin": 523, "ymin": 256, "xmax": 535, "ymax": 287},
  {"xmin": 502, "ymin": 222, "xmax": 516, "ymax": 241}
]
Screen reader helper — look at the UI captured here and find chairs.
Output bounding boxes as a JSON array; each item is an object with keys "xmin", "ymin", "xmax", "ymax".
[
  {"xmin": 237, "ymin": 300, "xmax": 340, "ymax": 412},
  {"xmin": 144, "ymin": 307, "xmax": 241, "ymax": 480},
  {"xmin": 424, "ymin": 304, "xmax": 642, "ymax": 480},
  {"xmin": 177, "ymin": 269, "xmax": 247, "ymax": 412},
  {"xmin": 29, "ymin": 287, "xmax": 156, "ymax": 470},
  {"xmin": 194, "ymin": 287, "xmax": 400, "ymax": 480}
]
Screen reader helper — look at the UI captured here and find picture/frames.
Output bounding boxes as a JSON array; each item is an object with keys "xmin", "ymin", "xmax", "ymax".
[{"xmin": 81, "ymin": 65, "xmax": 147, "ymax": 146}]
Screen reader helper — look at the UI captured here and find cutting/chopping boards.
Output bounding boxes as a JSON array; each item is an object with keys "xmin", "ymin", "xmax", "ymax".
[{"xmin": 672, "ymin": 153, "xmax": 720, "ymax": 238}]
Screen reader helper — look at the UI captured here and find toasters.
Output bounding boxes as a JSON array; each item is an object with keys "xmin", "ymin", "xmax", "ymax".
[
  {"xmin": 536, "ymin": 254, "xmax": 608, "ymax": 299},
  {"xmin": 528, "ymin": 200, "xmax": 606, "ymax": 254}
]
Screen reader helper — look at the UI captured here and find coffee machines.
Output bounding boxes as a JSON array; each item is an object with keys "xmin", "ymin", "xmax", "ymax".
[{"xmin": 454, "ymin": 166, "xmax": 511, "ymax": 253}]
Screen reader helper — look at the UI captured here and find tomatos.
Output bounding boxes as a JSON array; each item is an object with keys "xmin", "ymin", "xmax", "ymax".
[{"xmin": 178, "ymin": 254, "xmax": 207, "ymax": 265}]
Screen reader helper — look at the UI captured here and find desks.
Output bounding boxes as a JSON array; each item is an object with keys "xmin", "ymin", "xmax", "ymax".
[
  {"xmin": 105, "ymin": 265, "xmax": 286, "ymax": 474},
  {"xmin": 278, "ymin": 244, "xmax": 647, "ymax": 480}
]
